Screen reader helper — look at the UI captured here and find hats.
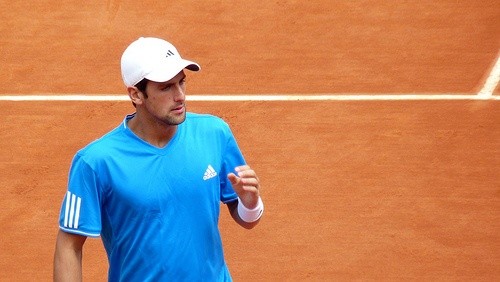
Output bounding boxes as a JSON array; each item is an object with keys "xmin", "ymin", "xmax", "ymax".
[{"xmin": 119, "ymin": 36, "xmax": 202, "ymax": 86}]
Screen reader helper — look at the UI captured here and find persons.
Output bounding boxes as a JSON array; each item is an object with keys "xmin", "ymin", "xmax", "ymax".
[{"xmin": 53, "ymin": 37, "xmax": 264, "ymax": 282}]
